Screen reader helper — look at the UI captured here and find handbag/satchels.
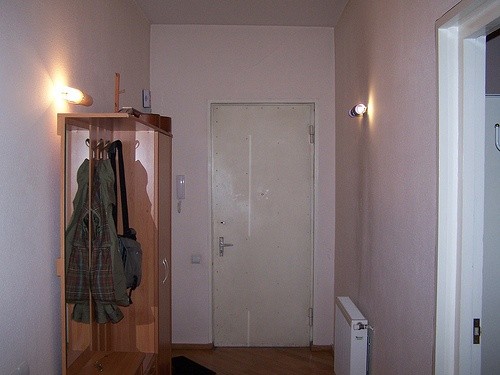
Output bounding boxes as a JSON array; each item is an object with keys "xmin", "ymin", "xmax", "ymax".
[{"xmin": 118, "ymin": 236, "xmax": 141, "ymax": 289}]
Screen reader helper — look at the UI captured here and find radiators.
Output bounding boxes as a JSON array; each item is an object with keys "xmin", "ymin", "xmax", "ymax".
[{"xmin": 333, "ymin": 295, "xmax": 372, "ymax": 375}]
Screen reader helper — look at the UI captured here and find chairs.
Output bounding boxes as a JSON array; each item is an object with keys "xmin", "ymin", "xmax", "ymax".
[
  {"xmin": 67, "ymin": 88, "xmax": 93, "ymax": 107},
  {"xmin": 348, "ymin": 103, "xmax": 367, "ymax": 117}
]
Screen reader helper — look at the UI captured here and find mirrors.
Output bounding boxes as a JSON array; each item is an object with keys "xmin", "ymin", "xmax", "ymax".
[{"xmin": 65, "ymin": 125, "xmax": 91, "ymax": 371}]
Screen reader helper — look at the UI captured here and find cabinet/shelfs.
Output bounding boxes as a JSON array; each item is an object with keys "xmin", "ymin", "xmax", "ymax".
[{"xmin": 56, "ymin": 112, "xmax": 175, "ymax": 375}]
